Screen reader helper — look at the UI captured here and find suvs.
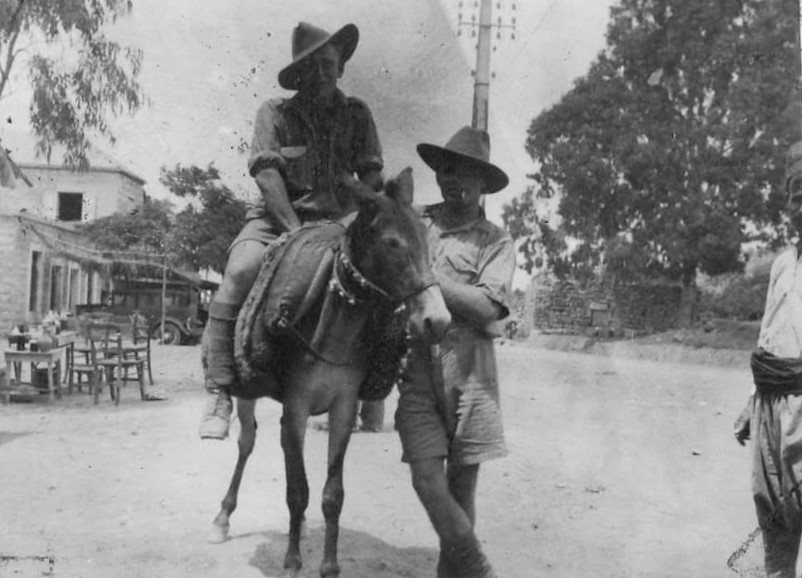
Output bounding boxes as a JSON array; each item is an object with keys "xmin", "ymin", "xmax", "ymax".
[{"xmin": 76, "ymin": 277, "xmax": 220, "ymax": 345}]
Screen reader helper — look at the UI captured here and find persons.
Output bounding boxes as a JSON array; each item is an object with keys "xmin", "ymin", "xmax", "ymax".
[
  {"xmin": 201, "ymin": 23, "xmax": 383, "ymax": 440},
  {"xmin": 406, "ymin": 126, "xmax": 515, "ymax": 578},
  {"xmin": 734, "ymin": 139, "xmax": 802, "ymax": 578}
]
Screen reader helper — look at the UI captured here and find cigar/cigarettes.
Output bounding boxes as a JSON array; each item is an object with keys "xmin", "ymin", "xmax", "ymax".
[{"xmin": 461, "ymin": 188, "xmax": 465, "ymax": 201}]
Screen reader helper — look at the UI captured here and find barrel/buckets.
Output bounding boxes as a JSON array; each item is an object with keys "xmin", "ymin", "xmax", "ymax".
[{"xmin": 31, "ymin": 342, "xmax": 62, "ymax": 387}]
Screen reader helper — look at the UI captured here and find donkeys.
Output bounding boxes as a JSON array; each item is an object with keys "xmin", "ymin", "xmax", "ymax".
[{"xmin": 207, "ymin": 165, "xmax": 451, "ymax": 578}]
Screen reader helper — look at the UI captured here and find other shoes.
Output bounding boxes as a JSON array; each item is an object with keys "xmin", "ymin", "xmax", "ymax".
[{"xmin": 200, "ymin": 391, "xmax": 233, "ymax": 439}]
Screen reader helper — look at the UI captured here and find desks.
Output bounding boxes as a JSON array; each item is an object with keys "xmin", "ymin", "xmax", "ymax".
[
  {"xmin": 8, "ymin": 330, "xmax": 77, "ymax": 387},
  {"xmin": 4, "ymin": 348, "xmax": 63, "ymax": 404}
]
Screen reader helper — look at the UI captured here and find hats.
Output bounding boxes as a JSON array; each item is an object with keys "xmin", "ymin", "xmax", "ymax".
[
  {"xmin": 784, "ymin": 140, "xmax": 801, "ymax": 179},
  {"xmin": 416, "ymin": 126, "xmax": 510, "ymax": 195},
  {"xmin": 277, "ymin": 20, "xmax": 360, "ymax": 91}
]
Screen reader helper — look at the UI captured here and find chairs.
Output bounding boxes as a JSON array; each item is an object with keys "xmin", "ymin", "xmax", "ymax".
[{"xmin": 63, "ymin": 313, "xmax": 154, "ymax": 404}]
